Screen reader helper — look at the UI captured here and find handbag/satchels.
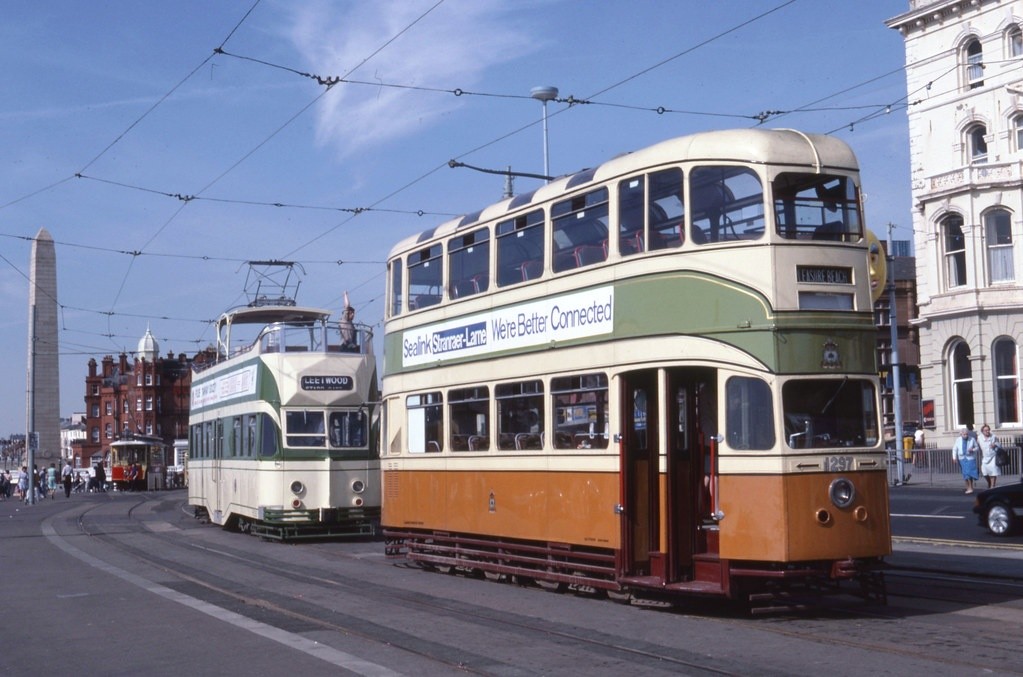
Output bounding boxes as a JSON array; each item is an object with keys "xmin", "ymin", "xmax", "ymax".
[
  {"xmin": 61, "ymin": 476, "xmax": 67, "ymax": 481},
  {"xmin": 996, "ymin": 448, "xmax": 1010, "ymax": 466}
]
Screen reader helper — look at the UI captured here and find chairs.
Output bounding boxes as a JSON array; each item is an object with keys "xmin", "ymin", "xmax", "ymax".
[
  {"xmin": 412, "ymin": 223, "xmax": 707, "ymax": 310},
  {"xmin": 426, "ymin": 431, "xmax": 600, "ymax": 452}
]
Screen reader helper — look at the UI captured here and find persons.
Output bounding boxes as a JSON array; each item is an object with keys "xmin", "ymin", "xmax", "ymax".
[
  {"xmin": 39, "ymin": 466, "xmax": 46, "ymax": 498},
  {"xmin": 76, "ymin": 472, "xmax": 84, "ymax": 492},
  {"xmin": 82, "ymin": 471, "xmax": 90, "ymax": 492},
  {"xmin": 912, "ymin": 424, "xmax": 924, "ymax": 465},
  {"xmin": 122, "ymin": 464, "xmax": 132, "ymax": 492},
  {"xmin": 952, "ymin": 428, "xmax": 979, "ymax": 494},
  {"xmin": 33, "ymin": 464, "xmax": 41, "ymax": 497},
  {"xmin": 130, "ymin": 463, "xmax": 138, "ymax": 492},
  {"xmin": 303, "ymin": 413, "xmax": 337, "ymax": 446},
  {"xmin": 0, "ymin": 470, "xmax": 12, "ymax": 498},
  {"xmin": 699, "ymin": 377, "xmax": 740, "ymax": 524},
  {"xmin": 62, "ymin": 461, "xmax": 75, "ymax": 497},
  {"xmin": 977, "ymin": 424, "xmax": 1001, "ymax": 489},
  {"xmin": 94, "ymin": 463, "xmax": 106, "ymax": 492},
  {"xmin": 46, "ymin": 463, "xmax": 58, "ymax": 499},
  {"xmin": 335, "ymin": 293, "xmax": 356, "ymax": 352},
  {"xmin": 17, "ymin": 466, "xmax": 28, "ymax": 502},
  {"xmin": 965, "ymin": 422, "xmax": 980, "ymax": 474}
]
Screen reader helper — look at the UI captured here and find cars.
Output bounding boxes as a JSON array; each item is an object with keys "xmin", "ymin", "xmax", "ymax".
[{"xmin": 973, "ymin": 478, "xmax": 1023, "ymax": 537}]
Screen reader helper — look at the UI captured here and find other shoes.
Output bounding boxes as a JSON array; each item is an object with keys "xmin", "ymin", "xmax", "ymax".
[{"xmin": 965, "ymin": 490, "xmax": 974, "ymax": 494}]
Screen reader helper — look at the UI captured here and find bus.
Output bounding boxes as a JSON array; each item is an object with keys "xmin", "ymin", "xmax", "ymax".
[
  {"xmin": 187, "ymin": 261, "xmax": 381, "ymax": 542},
  {"xmin": 109, "ymin": 390, "xmax": 168, "ymax": 491},
  {"xmin": 378, "ymin": 85, "xmax": 894, "ymax": 615}
]
